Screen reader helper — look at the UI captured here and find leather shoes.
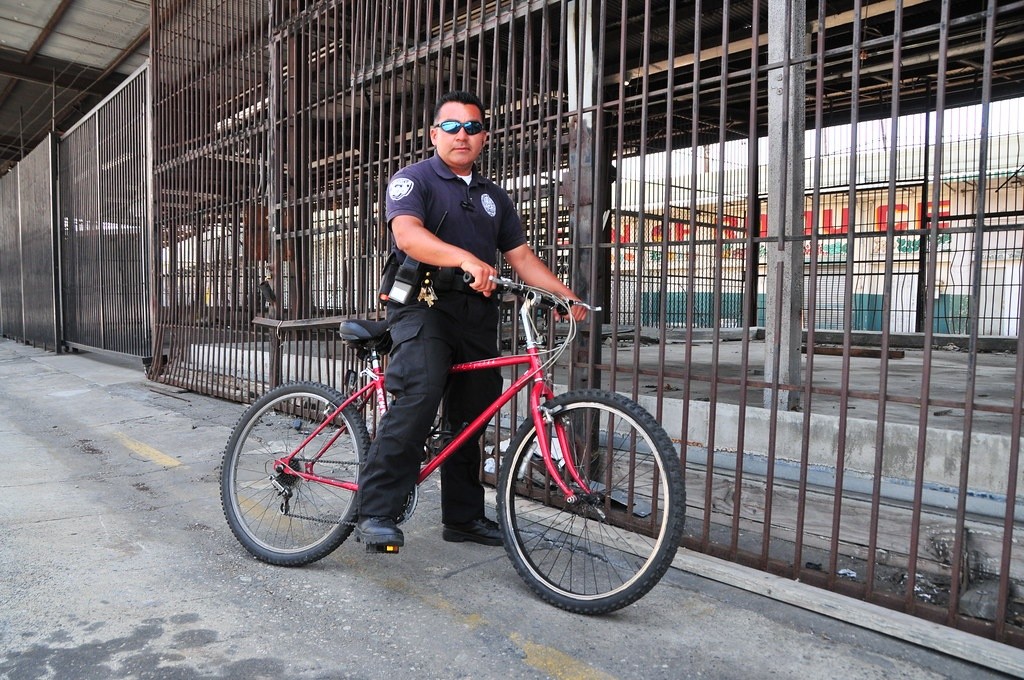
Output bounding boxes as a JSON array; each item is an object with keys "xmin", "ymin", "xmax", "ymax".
[
  {"xmin": 443, "ymin": 517, "xmax": 504, "ymax": 547},
  {"xmin": 353, "ymin": 515, "xmax": 404, "ymax": 548}
]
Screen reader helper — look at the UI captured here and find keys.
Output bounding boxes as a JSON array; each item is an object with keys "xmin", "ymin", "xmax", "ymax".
[{"xmin": 417, "ymin": 270, "xmax": 439, "ymax": 308}]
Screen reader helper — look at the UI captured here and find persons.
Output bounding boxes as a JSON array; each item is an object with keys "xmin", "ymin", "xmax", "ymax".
[{"xmin": 352, "ymin": 92, "xmax": 589, "ymax": 548}]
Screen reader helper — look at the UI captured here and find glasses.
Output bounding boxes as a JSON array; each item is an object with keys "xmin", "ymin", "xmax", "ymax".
[{"xmin": 433, "ymin": 119, "xmax": 485, "ymax": 136}]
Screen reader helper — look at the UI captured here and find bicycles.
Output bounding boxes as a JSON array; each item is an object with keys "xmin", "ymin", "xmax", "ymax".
[{"xmin": 221, "ymin": 276, "xmax": 688, "ymax": 616}]
